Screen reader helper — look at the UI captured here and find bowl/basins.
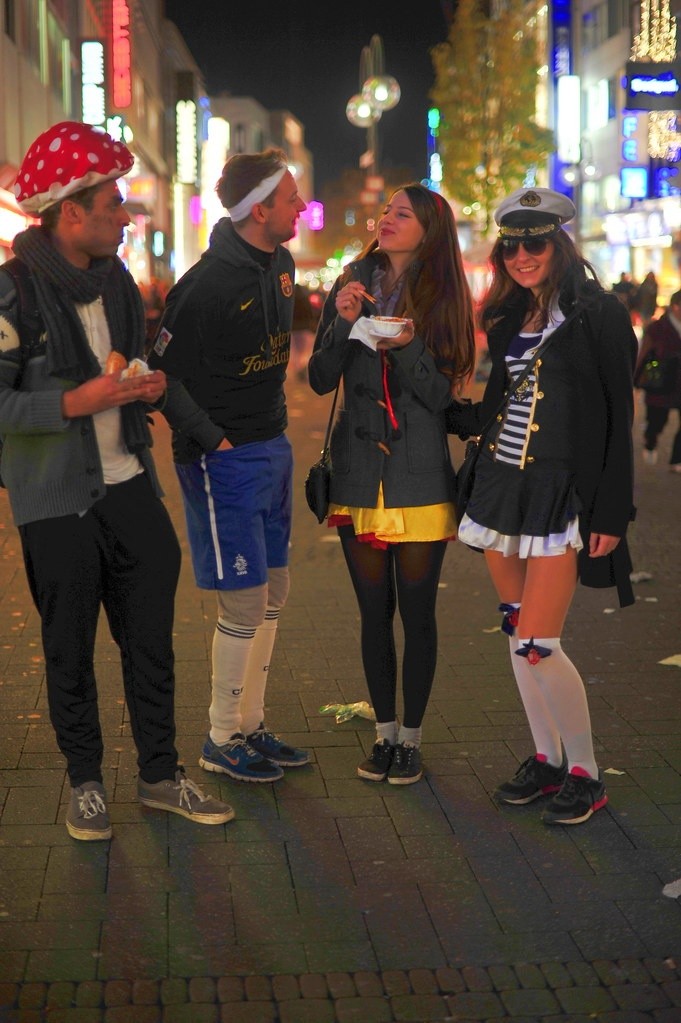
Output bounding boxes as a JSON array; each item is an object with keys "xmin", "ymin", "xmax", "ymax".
[{"xmin": 366, "ymin": 316, "xmax": 408, "ymax": 336}]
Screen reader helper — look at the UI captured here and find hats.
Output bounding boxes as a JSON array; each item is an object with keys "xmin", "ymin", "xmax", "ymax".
[
  {"xmin": 495, "ymin": 186, "xmax": 577, "ymax": 237},
  {"xmin": 13, "ymin": 121, "xmax": 135, "ymax": 216}
]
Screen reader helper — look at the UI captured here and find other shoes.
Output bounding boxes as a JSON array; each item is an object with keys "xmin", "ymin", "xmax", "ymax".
[
  {"xmin": 643, "ymin": 447, "xmax": 658, "ymax": 466},
  {"xmin": 670, "ymin": 463, "xmax": 681, "ymax": 475}
]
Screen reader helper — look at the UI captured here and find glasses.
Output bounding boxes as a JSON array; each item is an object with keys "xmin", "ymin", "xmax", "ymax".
[{"xmin": 501, "ymin": 235, "xmax": 554, "ymax": 261}]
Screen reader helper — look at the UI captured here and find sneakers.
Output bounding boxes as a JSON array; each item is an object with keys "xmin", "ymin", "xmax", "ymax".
[
  {"xmin": 136, "ymin": 769, "xmax": 234, "ymax": 825},
  {"xmin": 540, "ymin": 770, "xmax": 608, "ymax": 826},
  {"xmin": 358, "ymin": 737, "xmax": 395, "ymax": 780},
  {"xmin": 387, "ymin": 739, "xmax": 424, "ymax": 784},
  {"xmin": 491, "ymin": 755, "xmax": 569, "ymax": 804},
  {"xmin": 245, "ymin": 723, "xmax": 311, "ymax": 766},
  {"xmin": 199, "ymin": 732, "xmax": 284, "ymax": 783},
  {"xmin": 65, "ymin": 781, "xmax": 113, "ymax": 840}
]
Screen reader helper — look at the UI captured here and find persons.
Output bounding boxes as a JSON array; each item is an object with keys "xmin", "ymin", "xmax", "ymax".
[
  {"xmin": 0, "ymin": 121, "xmax": 236, "ymax": 839},
  {"xmin": 447, "ymin": 187, "xmax": 638, "ymax": 824},
  {"xmin": 308, "ymin": 180, "xmax": 475, "ymax": 785},
  {"xmin": 148, "ymin": 144, "xmax": 311, "ymax": 783},
  {"xmin": 598, "ymin": 270, "xmax": 680, "ymax": 474},
  {"xmin": 291, "ymin": 278, "xmax": 331, "ymax": 327},
  {"xmin": 135, "ymin": 275, "xmax": 178, "ymax": 338}
]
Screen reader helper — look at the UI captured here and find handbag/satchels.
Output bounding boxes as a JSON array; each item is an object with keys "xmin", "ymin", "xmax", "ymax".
[
  {"xmin": 304, "ymin": 455, "xmax": 331, "ymax": 525},
  {"xmin": 452, "ymin": 439, "xmax": 483, "ymax": 552},
  {"xmin": 637, "ymin": 356, "xmax": 665, "ymax": 391}
]
